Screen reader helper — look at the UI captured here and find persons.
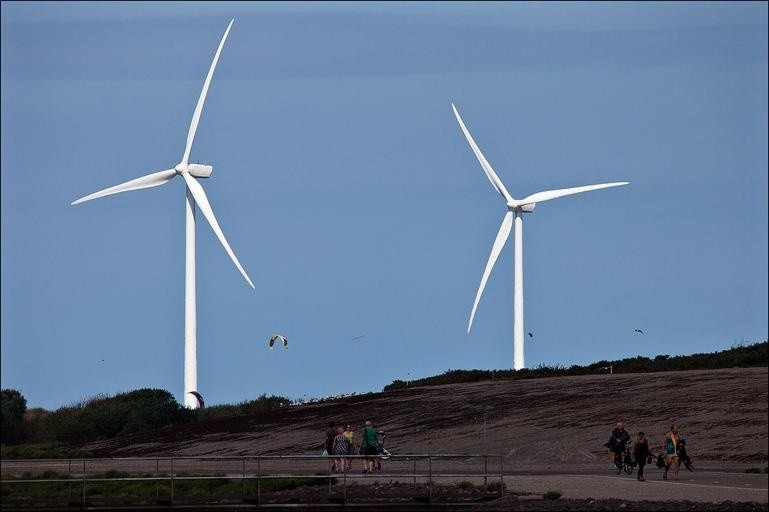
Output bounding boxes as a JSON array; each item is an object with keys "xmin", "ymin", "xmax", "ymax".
[
  {"xmin": 322, "ymin": 418, "xmax": 379, "ymax": 474},
  {"xmin": 660, "ymin": 422, "xmax": 683, "ymax": 481},
  {"xmin": 606, "ymin": 420, "xmax": 631, "ymax": 468},
  {"xmin": 630, "ymin": 430, "xmax": 652, "ymax": 482}
]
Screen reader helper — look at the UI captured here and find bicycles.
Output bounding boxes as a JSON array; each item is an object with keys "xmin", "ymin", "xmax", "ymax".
[{"xmin": 615, "ymin": 441, "xmax": 634, "ymax": 475}]
